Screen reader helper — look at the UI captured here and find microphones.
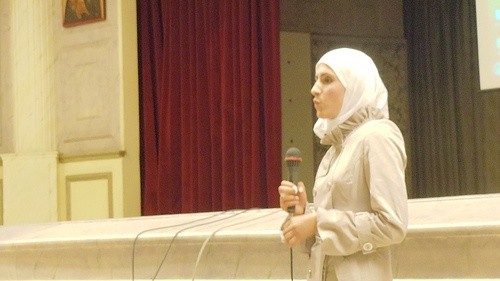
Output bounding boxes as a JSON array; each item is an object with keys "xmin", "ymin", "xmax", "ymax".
[{"xmin": 285, "ymin": 147, "xmax": 303, "ymax": 214}]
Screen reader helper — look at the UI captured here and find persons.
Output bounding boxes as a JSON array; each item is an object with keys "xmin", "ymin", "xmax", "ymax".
[{"xmin": 278, "ymin": 47, "xmax": 408, "ymax": 280}]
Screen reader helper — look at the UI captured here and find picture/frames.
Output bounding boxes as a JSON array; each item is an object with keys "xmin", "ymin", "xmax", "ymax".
[{"xmin": 61, "ymin": 0, "xmax": 106, "ymax": 28}]
